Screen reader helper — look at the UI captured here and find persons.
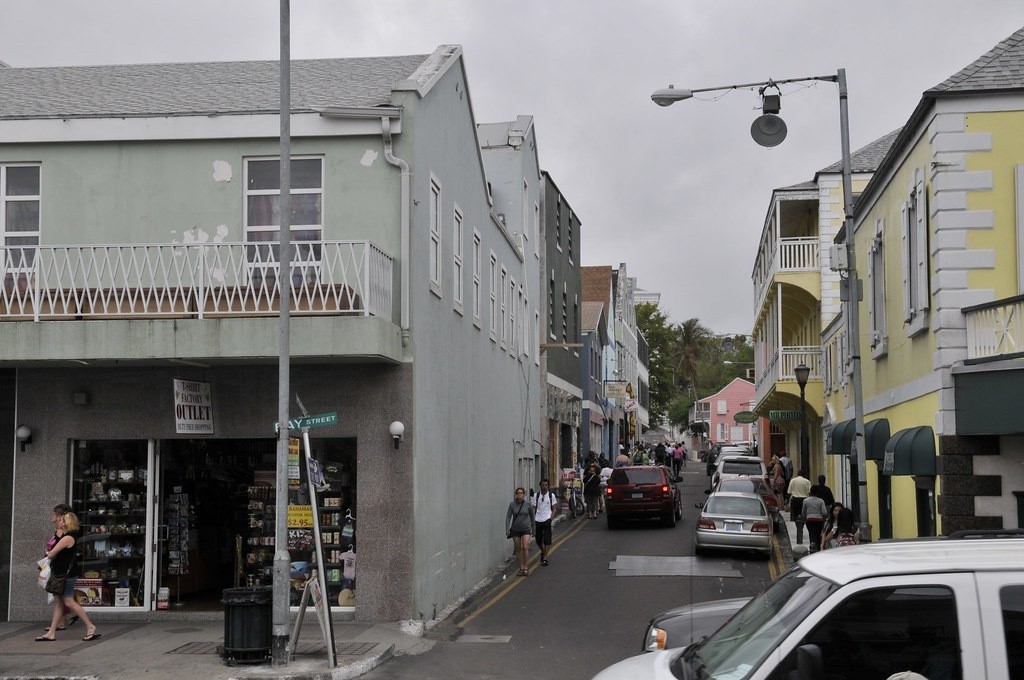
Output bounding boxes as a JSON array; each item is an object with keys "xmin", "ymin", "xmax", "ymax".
[
  {"xmin": 582, "ymin": 450, "xmax": 614, "ymax": 519},
  {"xmin": 786, "ymin": 470, "xmax": 812, "ymax": 526},
  {"xmin": 820, "ymin": 503, "xmax": 861, "ymax": 551},
  {"xmin": 768, "ymin": 450, "xmax": 793, "ymax": 509},
  {"xmin": 801, "ymin": 485, "xmax": 827, "ymax": 550},
  {"xmin": 615, "ymin": 438, "xmax": 653, "ymax": 467},
  {"xmin": 654, "ymin": 440, "xmax": 688, "ymax": 477},
  {"xmin": 506, "ymin": 487, "xmax": 536, "ymax": 576},
  {"xmin": 531, "ymin": 479, "xmax": 558, "ymax": 567},
  {"xmin": 816, "ymin": 475, "xmax": 835, "ymax": 513},
  {"xmin": 34, "ymin": 504, "xmax": 102, "ymax": 642}
]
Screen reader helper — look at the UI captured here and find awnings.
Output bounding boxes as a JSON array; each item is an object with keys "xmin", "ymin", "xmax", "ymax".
[
  {"xmin": 882, "ymin": 426, "xmax": 937, "ymax": 475},
  {"xmin": 850, "ymin": 418, "xmax": 890, "ymax": 464},
  {"xmin": 826, "ymin": 419, "xmax": 856, "ymax": 455}
]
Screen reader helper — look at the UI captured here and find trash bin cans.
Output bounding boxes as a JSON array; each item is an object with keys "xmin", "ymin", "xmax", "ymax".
[{"xmin": 223, "ymin": 588, "xmax": 272, "ymax": 666}]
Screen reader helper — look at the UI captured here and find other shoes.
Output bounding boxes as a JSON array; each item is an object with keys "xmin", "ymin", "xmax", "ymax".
[
  {"xmin": 523, "ymin": 569, "xmax": 528, "ymax": 576},
  {"xmin": 540, "ymin": 555, "xmax": 543, "ymax": 562},
  {"xmin": 598, "ymin": 509, "xmax": 603, "ymax": 514},
  {"xmin": 542, "ymin": 560, "xmax": 548, "ymax": 566},
  {"xmin": 517, "ymin": 570, "xmax": 523, "ymax": 575}
]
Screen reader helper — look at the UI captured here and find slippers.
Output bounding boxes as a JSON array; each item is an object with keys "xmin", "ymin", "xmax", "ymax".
[
  {"xmin": 35, "ymin": 635, "xmax": 56, "ymax": 641},
  {"xmin": 45, "ymin": 625, "xmax": 66, "ymax": 630},
  {"xmin": 69, "ymin": 616, "xmax": 78, "ymax": 625},
  {"xmin": 83, "ymin": 634, "xmax": 101, "ymax": 641}
]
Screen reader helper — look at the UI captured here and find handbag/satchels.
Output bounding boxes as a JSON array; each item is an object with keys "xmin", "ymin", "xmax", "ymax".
[
  {"xmin": 507, "ymin": 529, "xmax": 512, "ymax": 539},
  {"xmin": 38, "ymin": 557, "xmax": 52, "ymax": 589},
  {"xmin": 45, "ymin": 575, "xmax": 66, "ymax": 594}
]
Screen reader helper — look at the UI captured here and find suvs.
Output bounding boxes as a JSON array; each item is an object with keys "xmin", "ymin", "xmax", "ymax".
[
  {"xmin": 605, "ymin": 464, "xmax": 685, "ymax": 530},
  {"xmin": 591, "ymin": 529, "xmax": 1024, "ymax": 680}
]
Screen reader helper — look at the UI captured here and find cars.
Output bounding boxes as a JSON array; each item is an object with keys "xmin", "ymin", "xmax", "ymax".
[
  {"xmin": 698, "ymin": 441, "xmax": 772, "ymax": 487},
  {"xmin": 696, "ymin": 478, "xmax": 783, "ymax": 560}
]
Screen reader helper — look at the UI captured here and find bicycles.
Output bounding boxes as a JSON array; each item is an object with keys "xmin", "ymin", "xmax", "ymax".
[{"xmin": 568, "ymin": 487, "xmax": 585, "ymax": 519}]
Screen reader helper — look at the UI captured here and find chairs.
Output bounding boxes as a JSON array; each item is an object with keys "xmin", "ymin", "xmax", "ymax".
[{"xmin": 715, "ymin": 504, "xmax": 727, "ymax": 513}]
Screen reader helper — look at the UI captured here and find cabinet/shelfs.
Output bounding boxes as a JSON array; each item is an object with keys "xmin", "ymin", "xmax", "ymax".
[
  {"xmin": 69, "ymin": 460, "xmax": 147, "ymax": 607},
  {"xmin": 159, "ymin": 439, "xmax": 356, "ymax": 607}
]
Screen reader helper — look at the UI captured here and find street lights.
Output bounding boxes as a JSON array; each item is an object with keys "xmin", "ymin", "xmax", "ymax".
[
  {"xmin": 652, "ymin": 67, "xmax": 872, "ymax": 541},
  {"xmin": 795, "ymin": 360, "xmax": 812, "ymax": 479}
]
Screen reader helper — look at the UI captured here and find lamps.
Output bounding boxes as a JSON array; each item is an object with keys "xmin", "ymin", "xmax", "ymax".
[
  {"xmin": 389, "ymin": 421, "xmax": 405, "ymax": 449},
  {"xmin": 16, "ymin": 425, "xmax": 32, "ymax": 452}
]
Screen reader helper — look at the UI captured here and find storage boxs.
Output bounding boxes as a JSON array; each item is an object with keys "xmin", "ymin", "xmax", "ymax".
[
  {"xmin": 115, "ymin": 588, "xmax": 130, "ymax": 606},
  {"xmin": 72, "ymin": 579, "xmax": 103, "ymax": 606}
]
